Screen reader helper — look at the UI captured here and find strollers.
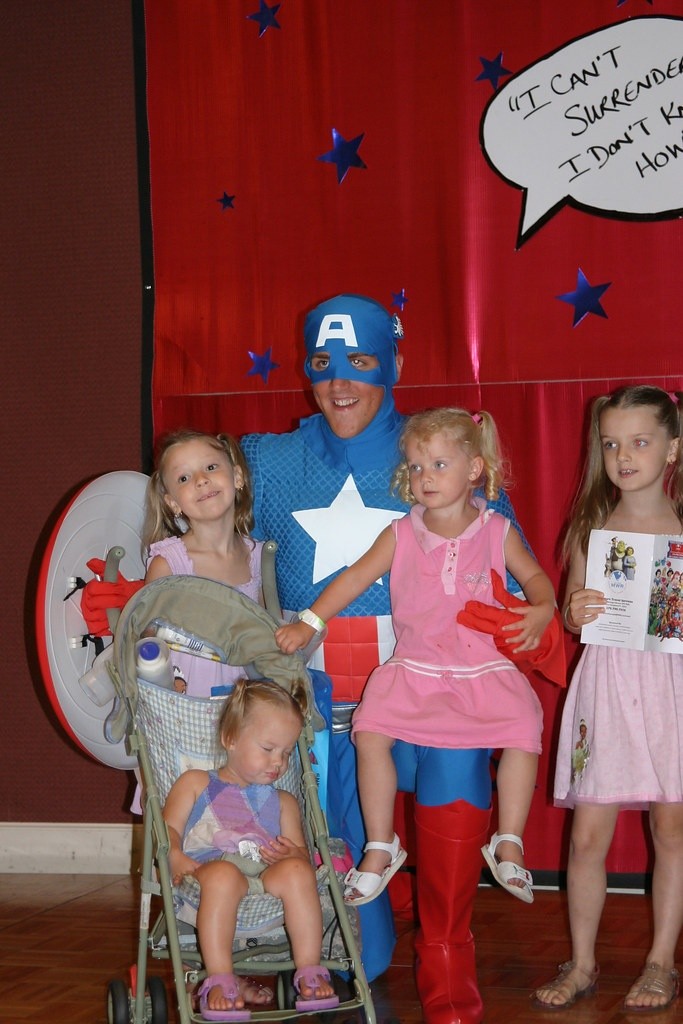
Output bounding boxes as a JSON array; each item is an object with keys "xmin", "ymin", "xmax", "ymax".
[{"xmin": 102, "ymin": 539, "xmax": 380, "ymax": 1023}]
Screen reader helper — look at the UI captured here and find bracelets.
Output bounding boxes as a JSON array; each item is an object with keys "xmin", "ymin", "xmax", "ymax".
[
  {"xmin": 299, "ymin": 608, "xmax": 326, "ymax": 636},
  {"xmin": 565, "ymin": 605, "xmax": 580, "ymax": 629}
]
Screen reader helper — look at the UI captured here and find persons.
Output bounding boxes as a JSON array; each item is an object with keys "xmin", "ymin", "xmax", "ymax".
[
  {"xmin": 535, "ymin": 386, "xmax": 683, "ymax": 1010},
  {"xmin": 161, "ymin": 678, "xmax": 339, "ymax": 1021},
  {"xmin": 274, "ymin": 408, "xmax": 555, "ymax": 903},
  {"xmin": 84, "ymin": 291, "xmax": 534, "ymax": 1024},
  {"xmin": 129, "ymin": 431, "xmax": 265, "ymax": 815}
]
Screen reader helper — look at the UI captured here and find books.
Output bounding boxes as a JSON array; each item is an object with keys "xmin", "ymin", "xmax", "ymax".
[{"xmin": 580, "ymin": 529, "xmax": 683, "ymax": 655}]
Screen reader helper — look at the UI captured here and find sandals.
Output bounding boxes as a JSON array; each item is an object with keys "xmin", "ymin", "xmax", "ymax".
[
  {"xmin": 529, "ymin": 961, "xmax": 600, "ymax": 1011},
  {"xmin": 294, "ymin": 966, "xmax": 339, "ymax": 1012},
  {"xmin": 624, "ymin": 962, "xmax": 681, "ymax": 1011},
  {"xmin": 234, "ymin": 974, "xmax": 275, "ymax": 1006},
  {"xmin": 480, "ymin": 831, "xmax": 534, "ymax": 903},
  {"xmin": 343, "ymin": 832, "xmax": 408, "ymax": 907},
  {"xmin": 196, "ymin": 974, "xmax": 251, "ymax": 1021}
]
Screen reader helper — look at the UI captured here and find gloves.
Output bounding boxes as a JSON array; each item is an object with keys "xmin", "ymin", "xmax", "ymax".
[
  {"xmin": 457, "ymin": 569, "xmax": 567, "ymax": 687},
  {"xmin": 80, "ymin": 559, "xmax": 145, "ymax": 638}
]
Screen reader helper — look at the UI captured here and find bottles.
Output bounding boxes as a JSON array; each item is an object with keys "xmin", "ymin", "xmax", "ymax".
[{"xmin": 79, "ymin": 637, "xmax": 174, "ymax": 707}]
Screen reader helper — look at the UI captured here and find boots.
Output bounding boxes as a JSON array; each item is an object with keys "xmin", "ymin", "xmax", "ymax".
[{"xmin": 413, "ymin": 793, "xmax": 494, "ymax": 1024}]
